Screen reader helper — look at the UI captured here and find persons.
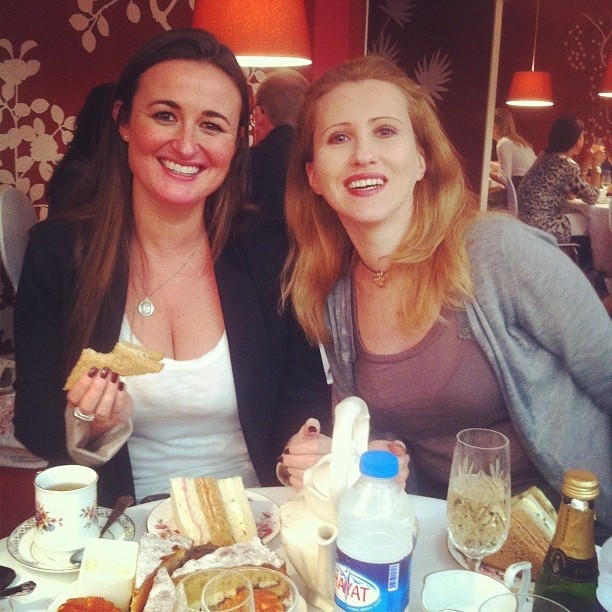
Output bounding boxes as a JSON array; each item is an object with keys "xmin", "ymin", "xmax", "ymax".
[
  {"xmin": 14, "ymin": 28, "xmax": 331, "ymax": 492},
  {"xmin": 487, "ymin": 109, "xmax": 537, "ymax": 213},
  {"xmin": 44, "ymin": 82, "xmax": 118, "ymax": 212},
  {"xmin": 281, "ymin": 56, "xmax": 612, "ymax": 547},
  {"xmin": 250, "ymin": 70, "xmax": 310, "ymax": 215},
  {"xmin": 517, "ymin": 117, "xmax": 607, "ymax": 270}
]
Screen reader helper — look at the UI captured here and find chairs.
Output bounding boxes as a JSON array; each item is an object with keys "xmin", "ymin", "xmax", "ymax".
[
  {"xmin": 0, "ymin": 183, "xmax": 39, "ymax": 294},
  {"xmin": 505, "ymin": 174, "xmax": 582, "ymax": 266}
]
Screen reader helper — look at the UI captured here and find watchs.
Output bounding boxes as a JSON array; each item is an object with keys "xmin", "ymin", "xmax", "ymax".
[{"xmin": 591, "ymin": 165, "xmax": 603, "ymax": 176}]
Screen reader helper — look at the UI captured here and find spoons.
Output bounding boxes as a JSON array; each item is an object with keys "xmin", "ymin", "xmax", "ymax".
[{"xmin": 70, "ymin": 495, "xmax": 135, "ymax": 568}]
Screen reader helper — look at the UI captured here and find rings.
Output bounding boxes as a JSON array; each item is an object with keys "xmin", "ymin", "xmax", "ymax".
[{"xmin": 74, "ymin": 408, "xmax": 94, "ymax": 425}]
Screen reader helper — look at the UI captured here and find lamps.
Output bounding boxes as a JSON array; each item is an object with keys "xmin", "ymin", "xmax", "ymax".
[
  {"xmin": 597, "ymin": 55, "xmax": 612, "ymax": 96},
  {"xmin": 189, "ymin": 0, "xmax": 313, "ymax": 66},
  {"xmin": 506, "ymin": 0, "xmax": 554, "ymax": 106}
]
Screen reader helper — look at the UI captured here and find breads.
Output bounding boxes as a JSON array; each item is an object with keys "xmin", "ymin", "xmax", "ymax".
[{"xmin": 62, "ymin": 340, "xmax": 166, "ymax": 391}]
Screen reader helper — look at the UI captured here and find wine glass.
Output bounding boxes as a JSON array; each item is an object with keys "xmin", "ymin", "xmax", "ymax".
[
  {"xmin": 446, "ymin": 428, "xmax": 511, "ymax": 573},
  {"xmin": 601, "ymin": 170, "xmax": 611, "ymax": 185}
]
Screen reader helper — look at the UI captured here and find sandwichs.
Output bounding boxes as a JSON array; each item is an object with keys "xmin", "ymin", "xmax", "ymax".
[
  {"xmin": 169, "ymin": 540, "xmax": 294, "ymax": 611},
  {"xmin": 169, "ymin": 474, "xmax": 261, "ymax": 548},
  {"xmin": 457, "ymin": 484, "xmax": 559, "ymax": 581}
]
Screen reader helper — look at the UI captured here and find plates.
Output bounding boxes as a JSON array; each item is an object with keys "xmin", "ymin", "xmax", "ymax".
[
  {"xmin": 146, "ymin": 490, "xmax": 282, "ymax": 556},
  {"xmin": 447, "ymin": 532, "xmax": 536, "ymax": 592},
  {"xmin": 292, "ymin": 591, "xmax": 307, "ymax": 612},
  {"xmin": 6, "ymin": 505, "xmax": 135, "ymax": 572}
]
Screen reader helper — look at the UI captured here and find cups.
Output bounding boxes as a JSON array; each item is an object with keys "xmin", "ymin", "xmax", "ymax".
[
  {"xmin": 597, "ymin": 189, "xmax": 608, "ymax": 203},
  {"xmin": 201, "ymin": 567, "xmax": 299, "ymax": 612},
  {"xmin": 421, "ymin": 561, "xmax": 532, "ymax": 612},
  {"xmin": 173, "ymin": 568, "xmax": 255, "ymax": 612},
  {"xmin": 33, "ymin": 464, "xmax": 100, "ymax": 552},
  {"xmin": 478, "ymin": 594, "xmax": 571, "ymax": 612}
]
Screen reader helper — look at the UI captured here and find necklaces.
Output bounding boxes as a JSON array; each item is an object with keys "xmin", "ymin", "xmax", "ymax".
[
  {"xmin": 129, "ymin": 227, "xmax": 214, "ymax": 316},
  {"xmin": 357, "ymin": 253, "xmax": 400, "ymax": 290}
]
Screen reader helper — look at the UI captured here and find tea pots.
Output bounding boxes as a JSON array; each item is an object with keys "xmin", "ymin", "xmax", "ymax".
[{"xmin": 280, "ymin": 397, "xmax": 418, "ymax": 612}]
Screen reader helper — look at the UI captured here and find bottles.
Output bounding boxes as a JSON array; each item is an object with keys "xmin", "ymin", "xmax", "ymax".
[
  {"xmin": 533, "ymin": 469, "xmax": 607, "ymax": 612},
  {"xmin": 333, "ymin": 451, "xmax": 419, "ymax": 612}
]
